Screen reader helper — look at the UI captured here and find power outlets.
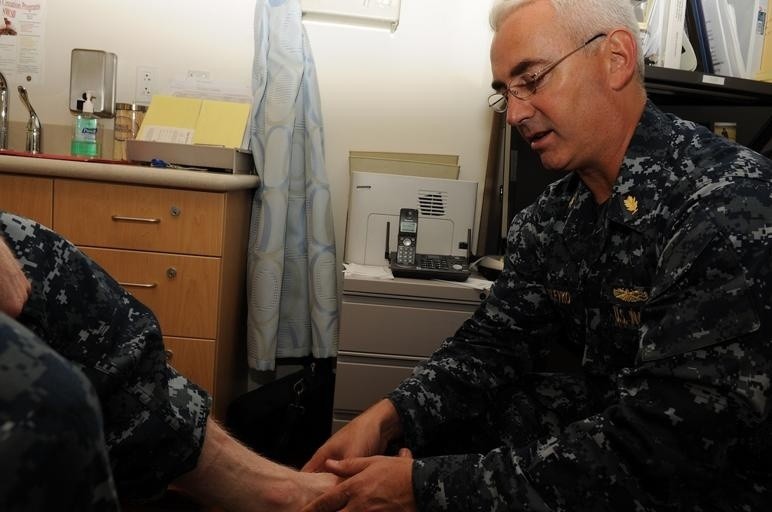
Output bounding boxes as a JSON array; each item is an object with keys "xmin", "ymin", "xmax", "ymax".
[{"xmin": 135, "ymin": 66, "xmax": 158, "ymax": 102}]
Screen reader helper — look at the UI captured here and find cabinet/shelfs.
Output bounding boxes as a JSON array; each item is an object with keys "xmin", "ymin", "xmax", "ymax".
[
  {"xmin": 1, "ymin": 173, "xmax": 261, "ymax": 423},
  {"xmin": 331, "ymin": 268, "xmax": 493, "ymax": 439}
]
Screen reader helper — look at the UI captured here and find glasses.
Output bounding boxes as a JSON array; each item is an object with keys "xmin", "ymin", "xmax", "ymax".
[{"xmin": 487, "ymin": 33, "xmax": 606, "ymax": 112}]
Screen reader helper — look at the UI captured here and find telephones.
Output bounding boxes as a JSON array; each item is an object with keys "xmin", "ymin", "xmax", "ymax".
[{"xmin": 384, "ymin": 207, "xmax": 472, "ymax": 280}]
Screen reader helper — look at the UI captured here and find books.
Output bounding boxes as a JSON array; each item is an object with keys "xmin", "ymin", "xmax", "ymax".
[
  {"xmin": 190, "ymin": 99, "xmax": 253, "ymax": 149},
  {"xmin": 133, "ymin": 93, "xmax": 204, "ymax": 147}
]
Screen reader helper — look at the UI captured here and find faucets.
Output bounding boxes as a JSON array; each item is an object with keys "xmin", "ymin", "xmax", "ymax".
[{"xmin": 1, "ymin": 70, "xmax": 9, "ymax": 147}]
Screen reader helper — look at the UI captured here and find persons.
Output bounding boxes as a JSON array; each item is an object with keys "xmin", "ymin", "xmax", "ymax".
[
  {"xmin": 0, "ymin": 210, "xmax": 347, "ymax": 511},
  {"xmin": 298, "ymin": 0, "xmax": 769, "ymax": 509}
]
